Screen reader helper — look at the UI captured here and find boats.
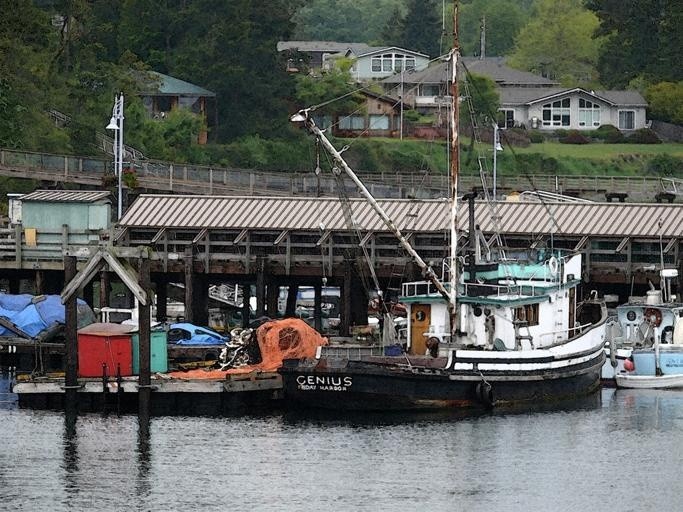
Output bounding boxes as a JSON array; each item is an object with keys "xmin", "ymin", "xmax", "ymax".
[
  {"xmin": 278, "ymin": 3, "xmax": 608, "ymax": 421},
  {"xmin": 588, "ymin": 268, "xmax": 682, "ymax": 391}
]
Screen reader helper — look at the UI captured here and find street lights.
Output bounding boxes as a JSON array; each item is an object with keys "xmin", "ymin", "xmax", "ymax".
[{"xmin": 106, "ymin": 94, "xmax": 124, "ymax": 222}]
